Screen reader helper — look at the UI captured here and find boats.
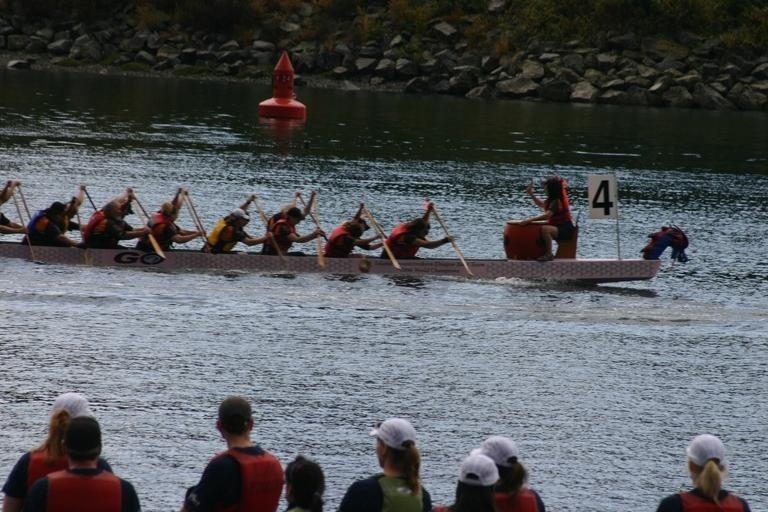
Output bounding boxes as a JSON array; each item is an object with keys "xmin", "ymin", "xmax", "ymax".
[{"xmin": 0, "ymin": 241, "xmax": 661, "ymax": 287}]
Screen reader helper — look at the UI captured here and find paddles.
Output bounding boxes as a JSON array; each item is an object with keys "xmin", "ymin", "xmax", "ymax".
[
  {"xmin": 130, "ymin": 202, "xmax": 168, "ymax": 259},
  {"xmin": 364, "ymin": 204, "xmax": 401, "ymax": 270},
  {"xmin": 314, "ymin": 195, "xmax": 326, "ymax": 268},
  {"xmin": 432, "ymin": 207, "xmax": 472, "ymax": 275},
  {"xmin": 254, "ymin": 200, "xmax": 288, "ymax": 265}
]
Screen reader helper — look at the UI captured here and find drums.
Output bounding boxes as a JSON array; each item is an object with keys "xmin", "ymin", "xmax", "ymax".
[{"xmin": 504, "ymin": 220, "xmax": 549, "ymax": 258}]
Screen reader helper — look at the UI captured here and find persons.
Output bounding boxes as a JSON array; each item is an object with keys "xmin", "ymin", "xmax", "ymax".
[
  {"xmin": 281, "ymin": 452, "xmax": 327, "ymax": 512},
  {"xmin": 338, "ymin": 417, "xmax": 432, "ymax": 512},
  {"xmin": 431, "ymin": 452, "xmax": 502, "ymax": 511},
  {"xmin": 654, "ymin": 433, "xmax": 751, "ymax": 512},
  {"xmin": 22, "ymin": 415, "xmax": 142, "ymax": 512},
  {"xmin": 518, "ymin": 174, "xmax": 575, "ymax": 262},
  {"xmin": 177, "ymin": 395, "xmax": 286, "ymax": 512},
  {"xmin": 0, "ymin": 390, "xmax": 115, "ymax": 512},
  {"xmin": 468, "ymin": 434, "xmax": 547, "ymax": 512}
]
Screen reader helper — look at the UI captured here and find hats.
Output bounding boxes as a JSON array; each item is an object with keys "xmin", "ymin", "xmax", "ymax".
[
  {"xmin": 125, "ymin": 203, "xmax": 134, "ymax": 214},
  {"xmin": 232, "ymin": 209, "xmax": 249, "ymax": 220},
  {"xmin": 286, "ymin": 208, "xmax": 305, "ymax": 220},
  {"xmin": 370, "ymin": 419, "xmax": 415, "ymax": 450},
  {"xmin": 542, "ymin": 178, "xmax": 565, "ymax": 187},
  {"xmin": 687, "ymin": 436, "xmax": 725, "ymax": 468},
  {"xmin": 459, "ymin": 456, "xmax": 500, "ymax": 485},
  {"xmin": 471, "ymin": 436, "xmax": 518, "ymax": 467},
  {"xmin": 54, "ymin": 394, "xmax": 92, "ymax": 419}
]
